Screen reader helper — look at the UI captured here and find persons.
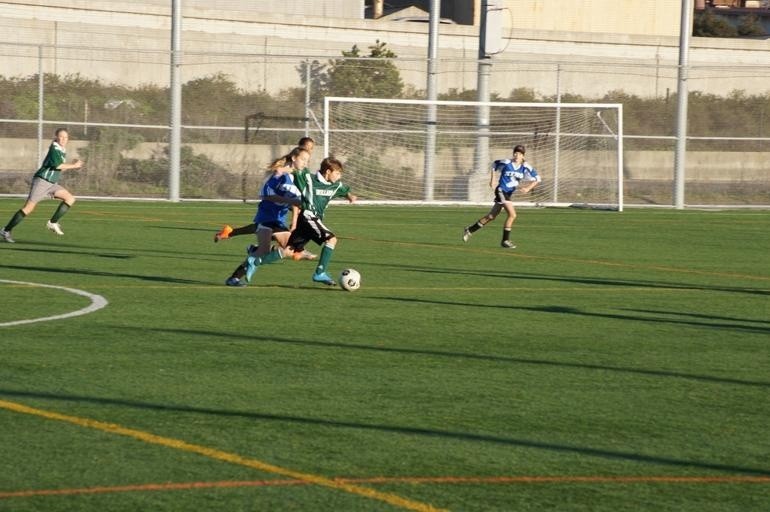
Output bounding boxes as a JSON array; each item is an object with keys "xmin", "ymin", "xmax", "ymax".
[
  {"xmin": 0, "ymin": 128, "xmax": 83, "ymax": 243},
  {"xmin": 247, "ymin": 156, "xmax": 356, "ymax": 285},
  {"xmin": 214, "ymin": 137, "xmax": 318, "ymax": 260},
  {"xmin": 225, "ymin": 148, "xmax": 310, "ymax": 286},
  {"xmin": 462, "ymin": 145, "xmax": 542, "ymax": 249}
]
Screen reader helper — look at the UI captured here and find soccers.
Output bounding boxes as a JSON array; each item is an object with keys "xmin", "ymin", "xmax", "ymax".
[{"xmin": 340, "ymin": 269, "xmax": 362, "ymax": 291}]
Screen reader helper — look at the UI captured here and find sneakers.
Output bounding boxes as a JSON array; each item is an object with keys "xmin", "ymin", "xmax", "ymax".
[
  {"xmin": 462, "ymin": 227, "xmax": 472, "ymax": 243},
  {"xmin": 293, "ymin": 248, "xmax": 318, "ymax": 261},
  {"xmin": 0, "ymin": 227, "xmax": 15, "ymax": 243},
  {"xmin": 214, "ymin": 225, "xmax": 233, "ymax": 243},
  {"xmin": 501, "ymin": 240, "xmax": 516, "ymax": 248},
  {"xmin": 245, "ymin": 242, "xmax": 257, "ymax": 283},
  {"xmin": 46, "ymin": 220, "xmax": 64, "ymax": 235},
  {"xmin": 312, "ymin": 272, "xmax": 337, "ymax": 286},
  {"xmin": 225, "ymin": 278, "xmax": 246, "ymax": 286}
]
